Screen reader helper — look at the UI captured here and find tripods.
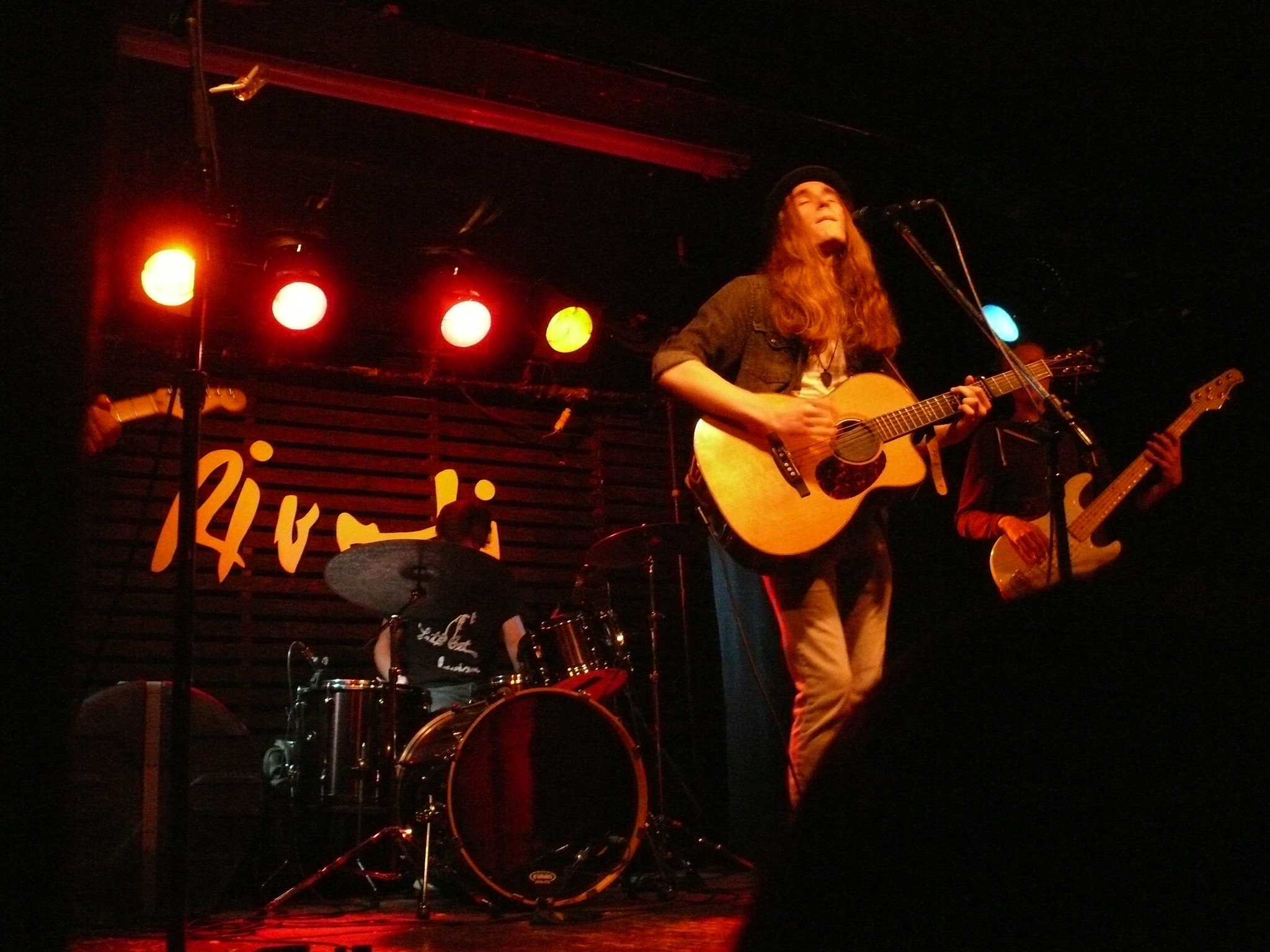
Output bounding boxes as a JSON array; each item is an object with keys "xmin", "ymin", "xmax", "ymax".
[
  {"xmin": 249, "ymin": 587, "xmax": 493, "ymax": 914},
  {"xmin": 643, "ymin": 554, "xmax": 761, "ymax": 876}
]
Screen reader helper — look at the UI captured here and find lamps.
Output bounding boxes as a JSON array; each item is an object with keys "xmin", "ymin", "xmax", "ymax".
[{"xmin": 126, "ymin": 237, "xmax": 594, "ymax": 365}]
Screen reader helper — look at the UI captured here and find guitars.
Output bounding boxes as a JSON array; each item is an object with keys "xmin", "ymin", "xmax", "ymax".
[
  {"xmin": 984, "ymin": 366, "xmax": 1244, "ymax": 604},
  {"xmin": 685, "ymin": 349, "xmax": 1099, "ymax": 573}
]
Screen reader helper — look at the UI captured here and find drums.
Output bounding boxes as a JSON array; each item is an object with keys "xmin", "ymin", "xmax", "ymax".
[{"xmin": 296, "ymin": 603, "xmax": 652, "ymax": 911}]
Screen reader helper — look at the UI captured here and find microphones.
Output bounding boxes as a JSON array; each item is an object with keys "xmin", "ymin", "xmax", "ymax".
[{"xmin": 849, "ymin": 195, "xmax": 938, "ymax": 226}]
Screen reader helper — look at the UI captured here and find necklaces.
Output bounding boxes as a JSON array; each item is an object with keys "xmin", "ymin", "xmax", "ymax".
[{"xmin": 812, "ymin": 338, "xmax": 840, "ymax": 387}]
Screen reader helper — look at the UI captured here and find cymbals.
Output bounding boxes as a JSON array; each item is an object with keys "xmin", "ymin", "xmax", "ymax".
[
  {"xmin": 323, "ymin": 538, "xmax": 522, "ymax": 616},
  {"xmin": 586, "ymin": 522, "xmax": 704, "ymax": 567}
]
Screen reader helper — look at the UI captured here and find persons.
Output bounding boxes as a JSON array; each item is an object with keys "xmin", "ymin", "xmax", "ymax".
[
  {"xmin": 954, "ymin": 342, "xmax": 1182, "ymax": 564},
  {"xmin": 652, "ymin": 165, "xmax": 992, "ymax": 815},
  {"xmin": 375, "ymin": 499, "xmax": 544, "ymax": 913}
]
